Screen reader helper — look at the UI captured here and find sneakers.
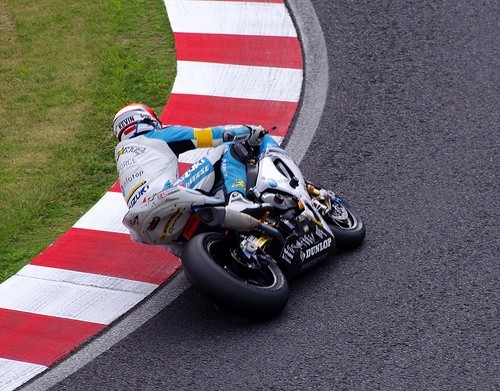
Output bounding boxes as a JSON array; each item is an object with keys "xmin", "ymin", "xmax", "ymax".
[{"xmin": 224, "ymin": 191, "xmax": 274, "ymax": 212}]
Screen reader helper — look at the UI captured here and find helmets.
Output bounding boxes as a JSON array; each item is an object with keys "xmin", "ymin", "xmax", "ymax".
[{"xmin": 113, "ymin": 102, "xmax": 163, "ymax": 142}]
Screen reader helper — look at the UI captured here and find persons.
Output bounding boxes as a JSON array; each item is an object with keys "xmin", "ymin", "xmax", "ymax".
[{"xmin": 112, "ymin": 102, "xmax": 274, "ymax": 221}]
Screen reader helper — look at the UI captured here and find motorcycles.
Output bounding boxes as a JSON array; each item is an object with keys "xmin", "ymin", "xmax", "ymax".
[{"xmin": 122, "ymin": 125, "xmax": 367, "ymax": 320}]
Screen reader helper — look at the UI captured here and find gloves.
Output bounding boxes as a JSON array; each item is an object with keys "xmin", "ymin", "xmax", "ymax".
[{"xmin": 246, "ymin": 124, "xmax": 265, "ymax": 146}]
